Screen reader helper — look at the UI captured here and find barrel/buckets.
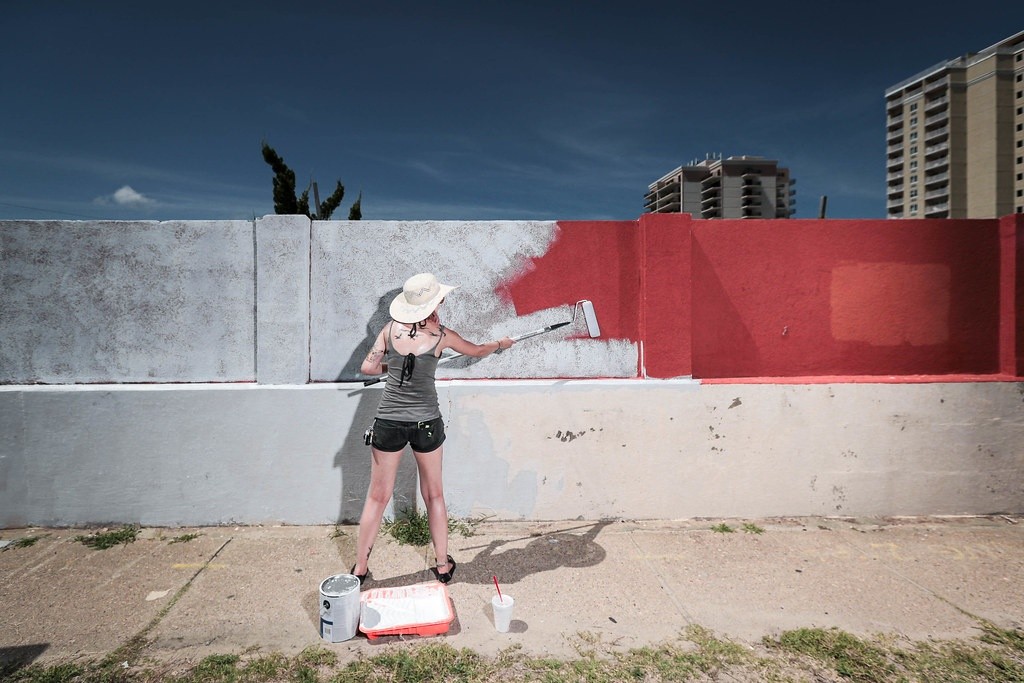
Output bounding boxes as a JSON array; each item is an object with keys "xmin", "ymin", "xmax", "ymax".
[{"xmin": 318, "ymin": 574, "xmax": 360, "ymax": 643}]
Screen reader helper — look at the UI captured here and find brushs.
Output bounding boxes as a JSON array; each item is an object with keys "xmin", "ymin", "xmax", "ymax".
[{"xmin": 364, "ymin": 299, "xmax": 601, "ymax": 390}]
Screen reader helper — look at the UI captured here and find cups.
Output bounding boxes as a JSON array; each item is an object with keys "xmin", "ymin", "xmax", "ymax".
[{"xmin": 492, "ymin": 595, "xmax": 514, "ymax": 632}]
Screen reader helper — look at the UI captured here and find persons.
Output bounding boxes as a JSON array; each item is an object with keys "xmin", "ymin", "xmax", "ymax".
[{"xmin": 350, "ymin": 273, "xmax": 517, "ymax": 586}]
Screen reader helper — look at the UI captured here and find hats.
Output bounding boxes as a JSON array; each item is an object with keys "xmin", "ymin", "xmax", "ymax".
[{"xmin": 389, "ymin": 273, "xmax": 454, "ymax": 323}]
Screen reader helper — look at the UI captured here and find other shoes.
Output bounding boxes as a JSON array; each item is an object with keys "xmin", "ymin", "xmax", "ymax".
[
  {"xmin": 350, "ymin": 564, "xmax": 368, "ymax": 586},
  {"xmin": 438, "ymin": 554, "xmax": 456, "ymax": 583}
]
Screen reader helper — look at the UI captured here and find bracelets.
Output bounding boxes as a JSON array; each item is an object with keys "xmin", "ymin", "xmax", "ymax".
[{"xmin": 493, "ymin": 340, "xmax": 502, "ymax": 356}]
